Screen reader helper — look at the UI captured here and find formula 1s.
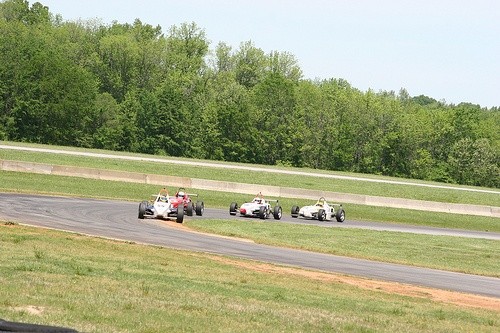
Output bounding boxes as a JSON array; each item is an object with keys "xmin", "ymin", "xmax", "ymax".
[
  {"xmin": 229, "ymin": 192, "xmax": 282, "ymax": 220},
  {"xmin": 291, "ymin": 196, "xmax": 345, "ymax": 222},
  {"xmin": 138, "ymin": 187, "xmax": 184, "ymax": 223},
  {"xmin": 169, "ymin": 187, "xmax": 205, "ymax": 216}
]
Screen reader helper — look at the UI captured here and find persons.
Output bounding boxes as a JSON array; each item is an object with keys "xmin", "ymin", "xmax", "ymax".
[
  {"xmin": 316, "ymin": 202, "xmax": 324, "ymax": 207},
  {"xmin": 158, "ymin": 195, "xmax": 168, "ymax": 203},
  {"xmin": 177, "ymin": 191, "xmax": 185, "ymax": 200},
  {"xmin": 254, "ymin": 198, "xmax": 262, "ymax": 204}
]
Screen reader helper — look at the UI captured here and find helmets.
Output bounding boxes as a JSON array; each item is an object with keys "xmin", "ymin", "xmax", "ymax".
[
  {"xmin": 255, "ymin": 198, "xmax": 260, "ymax": 203},
  {"xmin": 178, "ymin": 192, "xmax": 185, "ymax": 199},
  {"xmin": 160, "ymin": 195, "xmax": 167, "ymax": 202}
]
